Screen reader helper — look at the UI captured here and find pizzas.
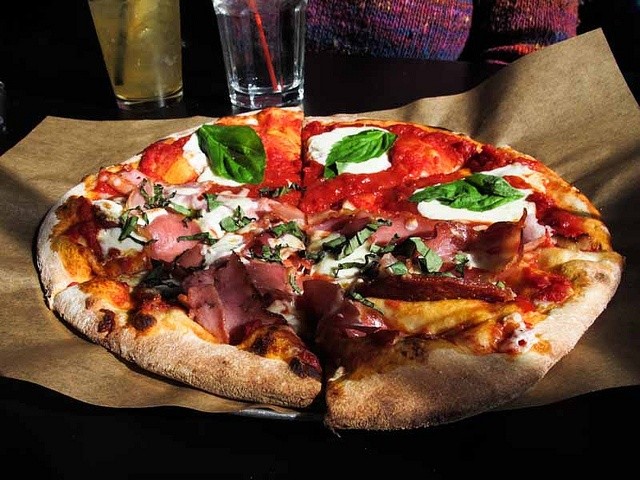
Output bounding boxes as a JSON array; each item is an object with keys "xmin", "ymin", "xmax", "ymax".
[{"xmin": 35, "ymin": 105, "xmax": 626, "ymax": 433}]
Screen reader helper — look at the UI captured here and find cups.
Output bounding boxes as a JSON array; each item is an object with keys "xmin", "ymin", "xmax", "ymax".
[
  {"xmin": 212, "ymin": 0, "xmax": 309, "ymax": 118},
  {"xmin": 87, "ymin": 0, "xmax": 186, "ymax": 113}
]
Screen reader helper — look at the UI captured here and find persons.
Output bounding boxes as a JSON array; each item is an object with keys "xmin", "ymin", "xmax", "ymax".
[{"xmin": 221, "ymin": 1, "xmax": 580, "ymax": 66}]
